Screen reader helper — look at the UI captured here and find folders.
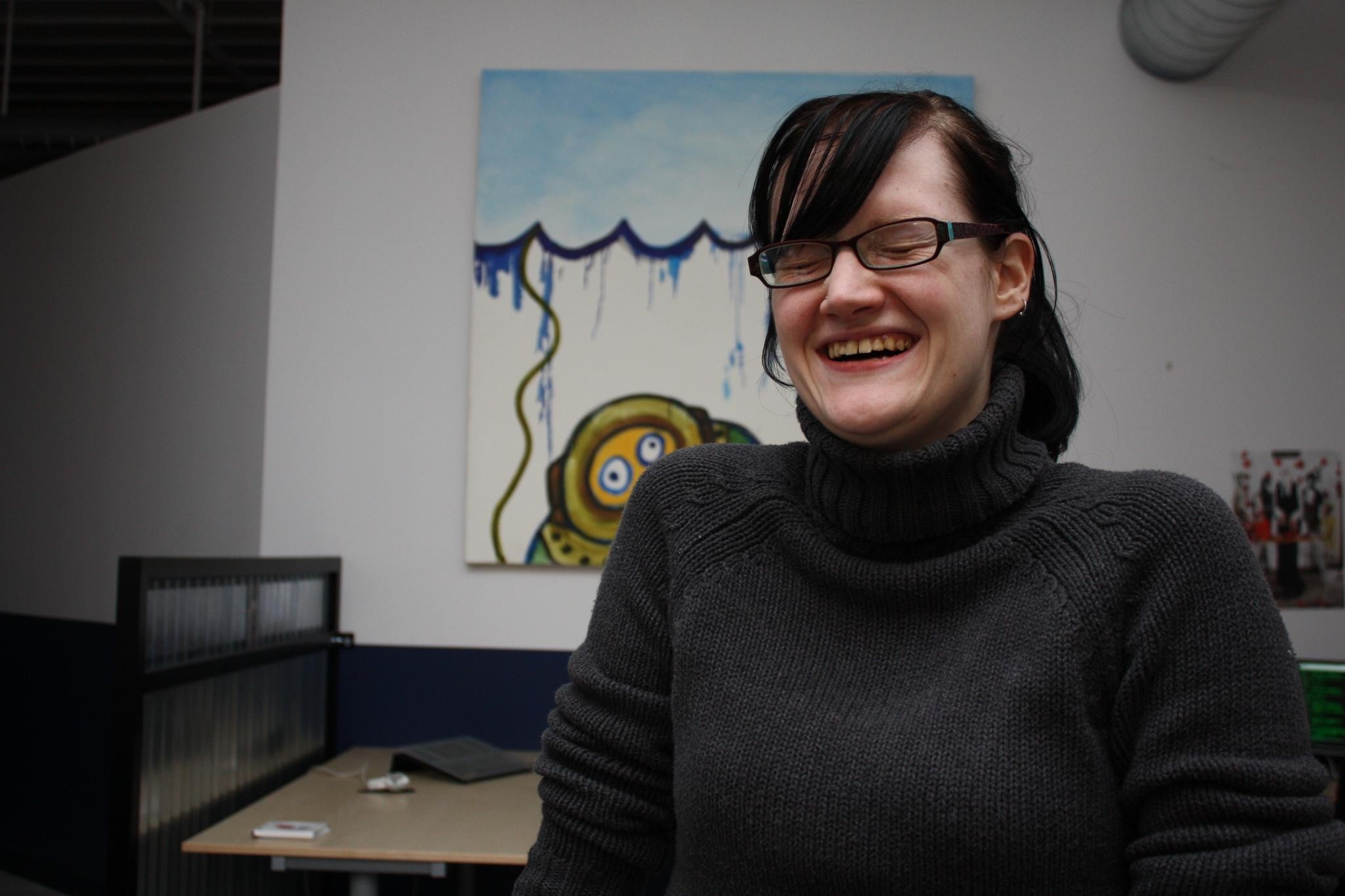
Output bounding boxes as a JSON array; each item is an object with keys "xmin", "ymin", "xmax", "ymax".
[{"xmin": 390, "ymin": 736, "xmax": 534, "ymax": 784}]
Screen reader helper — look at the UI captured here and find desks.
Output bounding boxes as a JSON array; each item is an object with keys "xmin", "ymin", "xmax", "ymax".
[{"xmin": 180, "ymin": 746, "xmax": 541, "ymax": 895}]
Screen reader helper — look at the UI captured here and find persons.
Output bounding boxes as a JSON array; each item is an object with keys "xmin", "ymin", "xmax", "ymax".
[{"xmin": 516, "ymin": 91, "xmax": 1345, "ymax": 895}]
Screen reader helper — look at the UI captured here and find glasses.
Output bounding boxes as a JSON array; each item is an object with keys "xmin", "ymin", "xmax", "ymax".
[{"xmin": 747, "ymin": 216, "xmax": 1018, "ymax": 289}]
